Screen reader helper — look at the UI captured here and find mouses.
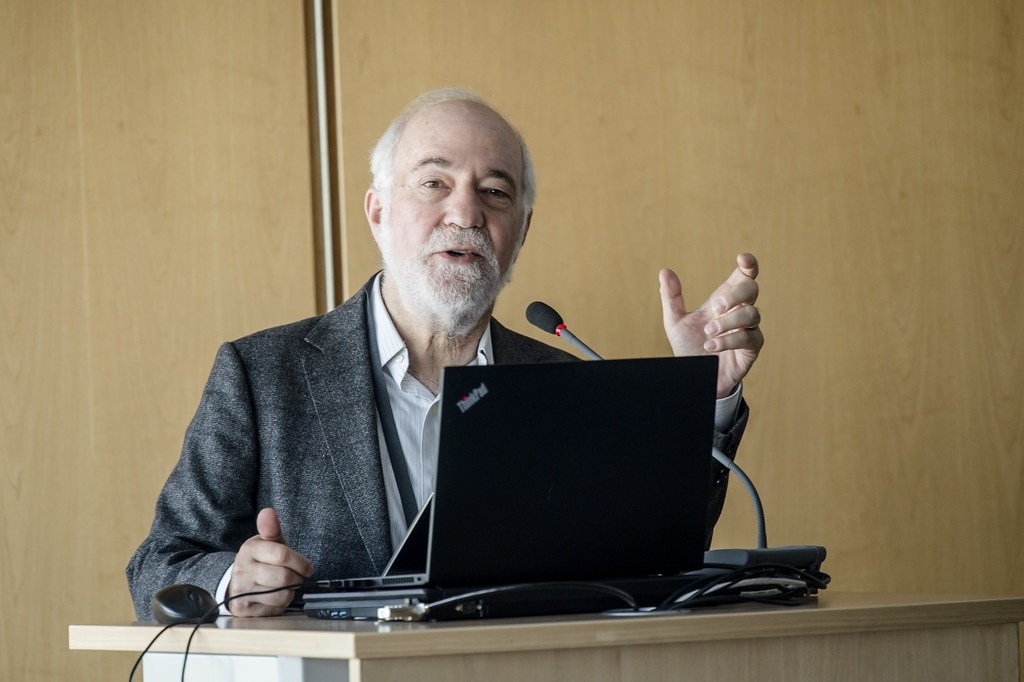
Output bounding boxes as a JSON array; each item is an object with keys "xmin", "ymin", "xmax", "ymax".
[{"xmin": 151, "ymin": 583, "xmax": 219, "ymax": 627}]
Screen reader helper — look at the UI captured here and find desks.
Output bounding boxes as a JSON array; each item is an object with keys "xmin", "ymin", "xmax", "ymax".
[{"xmin": 69, "ymin": 592, "xmax": 1024, "ymax": 682}]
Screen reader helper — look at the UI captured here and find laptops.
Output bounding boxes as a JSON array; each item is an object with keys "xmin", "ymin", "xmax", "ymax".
[{"xmin": 300, "ymin": 354, "xmax": 720, "ymax": 621}]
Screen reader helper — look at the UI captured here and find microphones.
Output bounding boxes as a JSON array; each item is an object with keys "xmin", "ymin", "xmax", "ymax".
[{"xmin": 526, "ymin": 299, "xmax": 826, "ymax": 589}]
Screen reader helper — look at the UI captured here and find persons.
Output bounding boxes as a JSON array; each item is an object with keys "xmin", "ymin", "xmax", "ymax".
[{"xmin": 124, "ymin": 89, "xmax": 765, "ymax": 626}]
